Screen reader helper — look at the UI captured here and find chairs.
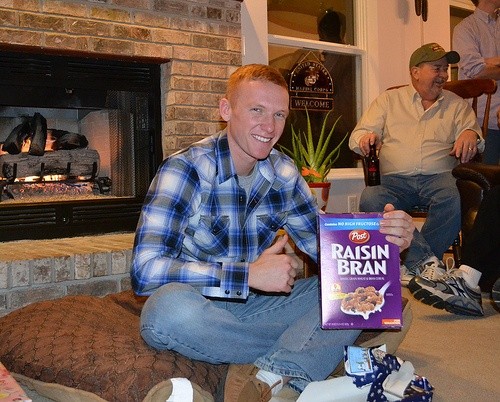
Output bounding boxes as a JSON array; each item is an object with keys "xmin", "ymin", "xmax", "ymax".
[{"xmin": 362, "ymin": 78, "xmax": 498, "ymax": 270}]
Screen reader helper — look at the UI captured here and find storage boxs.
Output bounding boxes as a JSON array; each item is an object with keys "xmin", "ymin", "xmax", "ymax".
[{"xmin": 314, "ymin": 209, "xmax": 404, "ymax": 330}]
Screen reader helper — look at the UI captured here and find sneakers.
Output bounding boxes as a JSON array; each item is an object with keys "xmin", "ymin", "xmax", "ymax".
[
  {"xmin": 409, "ymin": 275, "xmax": 485, "ymax": 316},
  {"xmin": 400, "ymin": 260, "xmax": 445, "ymax": 286},
  {"xmin": 215, "ymin": 363, "xmax": 281, "ymax": 402},
  {"xmin": 492, "ymin": 279, "xmax": 500, "ymax": 310}
]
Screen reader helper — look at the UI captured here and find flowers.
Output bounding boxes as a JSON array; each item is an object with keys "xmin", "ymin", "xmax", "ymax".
[{"xmin": 278, "ymin": 103, "xmax": 351, "ymax": 181}]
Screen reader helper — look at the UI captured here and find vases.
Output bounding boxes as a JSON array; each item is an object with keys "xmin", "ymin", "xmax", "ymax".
[{"xmin": 308, "ymin": 182, "xmax": 332, "ymax": 213}]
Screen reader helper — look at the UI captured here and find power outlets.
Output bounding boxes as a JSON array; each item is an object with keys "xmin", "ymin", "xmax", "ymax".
[{"xmin": 347, "ymin": 196, "xmax": 358, "ymax": 213}]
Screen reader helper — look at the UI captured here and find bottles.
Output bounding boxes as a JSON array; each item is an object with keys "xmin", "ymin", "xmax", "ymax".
[{"xmin": 368, "ymin": 145, "xmax": 381, "ymax": 188}]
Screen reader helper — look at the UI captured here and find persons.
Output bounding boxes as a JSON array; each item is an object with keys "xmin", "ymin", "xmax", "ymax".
[
  {"xmin": 129, "ymin": 64, "xmax": 416, "ymax": 402},
  {"xmin": 452, "ymin": 0, "xmax": 500, "ymax": 164},
  {"xmin": 349, "ymin": 43, "xmax": 483, "ymax": 288},
  {"xmin": 408, "ymin": 184, "xmax": 500, "ymax": 317}
]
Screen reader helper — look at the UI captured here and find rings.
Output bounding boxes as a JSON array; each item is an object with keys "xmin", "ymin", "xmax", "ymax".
[{"xmin": 468, "ymin": 148, "xmax": 473, "ymax": 150}]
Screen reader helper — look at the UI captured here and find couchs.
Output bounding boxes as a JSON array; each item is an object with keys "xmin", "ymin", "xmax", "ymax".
[{"xmin": 453, "ymin": 150, "xmax": 500, "ymax": 224}]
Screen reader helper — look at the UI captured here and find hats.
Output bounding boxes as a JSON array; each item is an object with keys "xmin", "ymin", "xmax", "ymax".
[{"xmin": 410, "ymin": 43, "xmax": 460, "ymax": 74}]
